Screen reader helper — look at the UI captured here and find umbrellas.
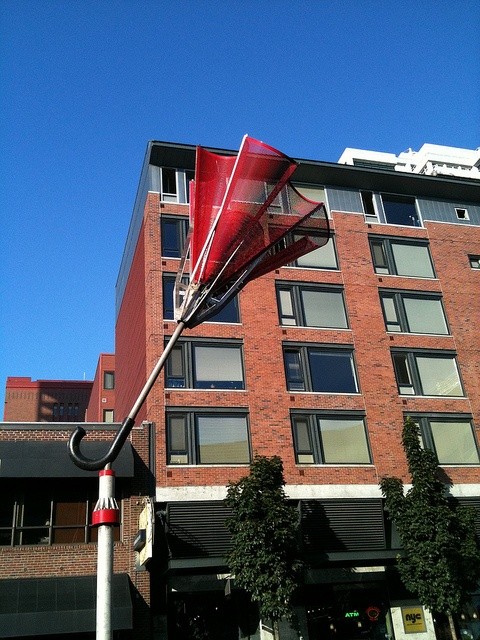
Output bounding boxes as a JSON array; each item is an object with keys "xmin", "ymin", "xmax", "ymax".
[{"xmin": 67, "ymin": 133, "xmax": 329, "ymax": 471}]
